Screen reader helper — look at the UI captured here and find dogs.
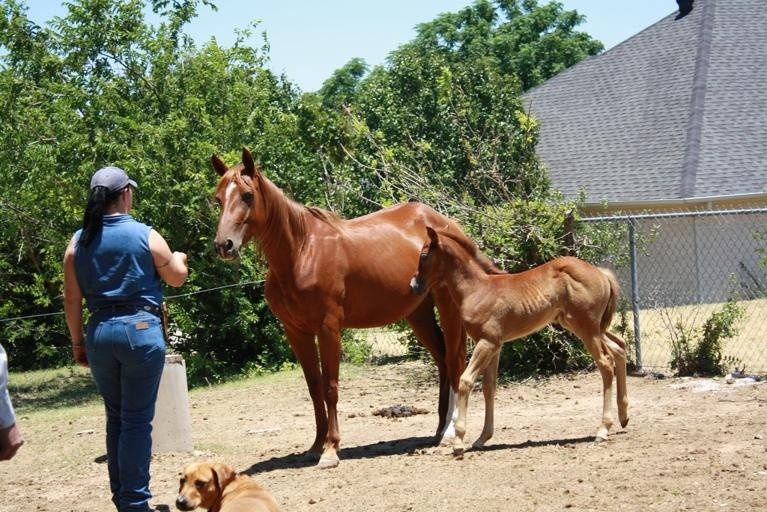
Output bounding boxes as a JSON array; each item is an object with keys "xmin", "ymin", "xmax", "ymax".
[{"xmin": 172, "ymin": 460, "xmax": 280, "ymax": 512}]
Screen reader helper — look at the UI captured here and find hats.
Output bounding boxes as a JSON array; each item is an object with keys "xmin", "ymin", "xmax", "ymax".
[{"xmin": 90, "ymin": 166, "xmax": 138, "ymax": 194}]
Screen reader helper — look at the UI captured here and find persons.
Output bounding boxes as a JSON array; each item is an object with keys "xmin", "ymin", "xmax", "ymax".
[
  {"xmin": 0, "ymin": 343, "xmax": 24, "ymax": 460},
  {"xmin": 63, "ymin": 166, "xmax": 188, "ymax": 512}
]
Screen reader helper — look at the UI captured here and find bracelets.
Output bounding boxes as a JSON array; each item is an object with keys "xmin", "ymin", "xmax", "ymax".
[{"xmin": 72, "ymin": 337, "xmax": 87, "ymax": 348}]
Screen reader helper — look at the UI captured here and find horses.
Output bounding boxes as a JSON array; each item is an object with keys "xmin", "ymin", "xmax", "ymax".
[
  {"xmin": 205, "ymin": 146, "xmax": 468, "ymax": 473},
  {"xmin": 407, "ymin": 224, "xmax": 630, "ymax": 459}
]
